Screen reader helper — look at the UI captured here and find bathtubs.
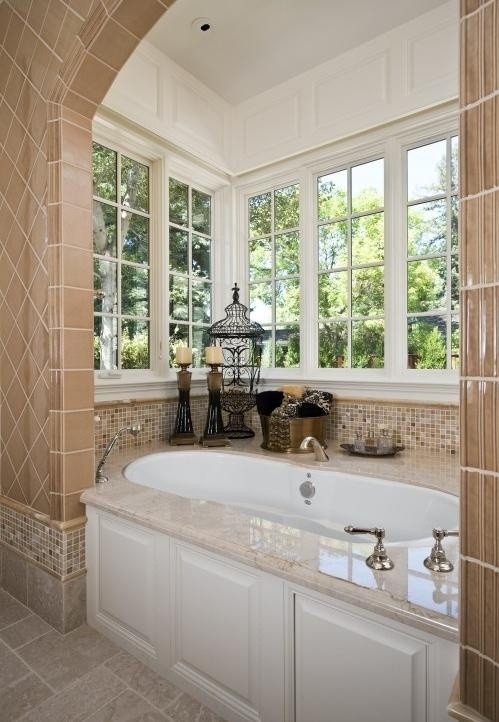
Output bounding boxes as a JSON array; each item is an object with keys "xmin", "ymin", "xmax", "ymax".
[{"xmin": 117, "ymin": 444, "xmax": 461, "ymax": 550}]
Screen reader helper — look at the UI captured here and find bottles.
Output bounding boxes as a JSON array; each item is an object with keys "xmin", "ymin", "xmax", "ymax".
[
  {"xmin": 376, "ymin": 428, "xmax": 394, "ymax": 455},
  {"xmin": 354, "ymin": 431, "xmax": 365, "ymax": 453}
]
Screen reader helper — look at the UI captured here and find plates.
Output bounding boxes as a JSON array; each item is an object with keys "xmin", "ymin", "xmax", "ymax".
[{"xmin": 340, "ymin": 444, "xmax": 405, "ymax": 458}]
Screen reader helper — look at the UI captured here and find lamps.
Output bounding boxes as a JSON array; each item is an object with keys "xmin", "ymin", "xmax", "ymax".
[{"xmin": 208, "ymin": 283, "xmax": 265, "ymax": 439}]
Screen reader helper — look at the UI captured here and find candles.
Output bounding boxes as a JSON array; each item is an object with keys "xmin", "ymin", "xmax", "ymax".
[
  {"xmin": 205, "ymin": 347, "xmax": 222, "ymax": 364},
  {"xmin": 175, "ymin": 347, "xmax": 192, "ymax": 363}
]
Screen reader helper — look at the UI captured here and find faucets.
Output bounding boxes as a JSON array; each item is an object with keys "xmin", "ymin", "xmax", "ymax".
[{"xmin": 300, "ymin": 434, "xmax": 327, "ymax": 461}]
